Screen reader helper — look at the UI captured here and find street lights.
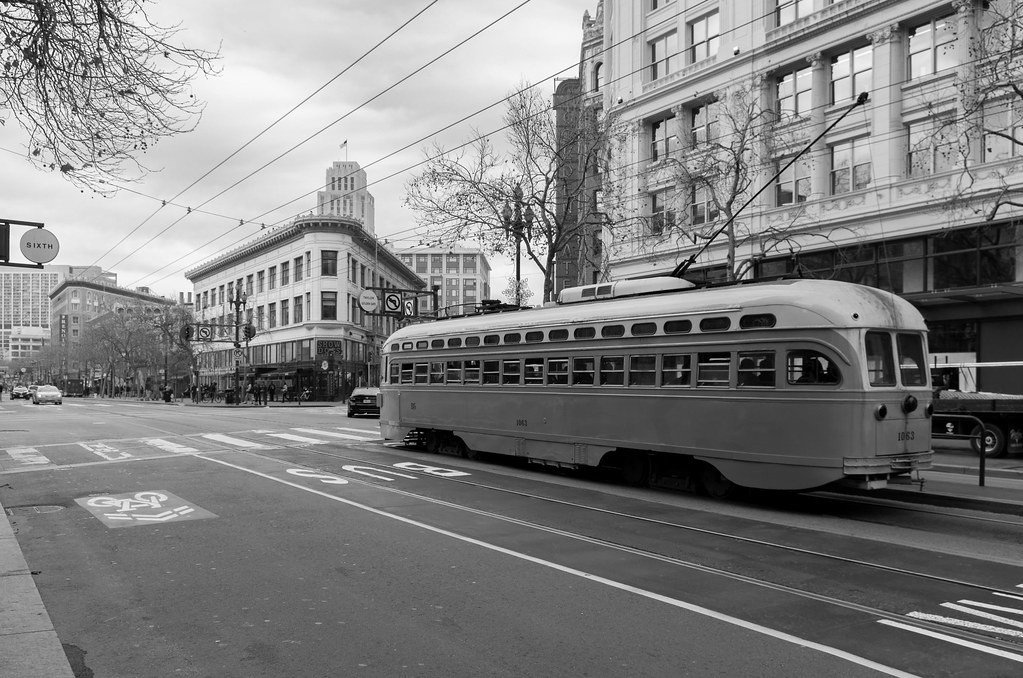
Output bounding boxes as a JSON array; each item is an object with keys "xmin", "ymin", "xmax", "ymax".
[
  {"xmin": 154, "ymin": 323, "xmax": 168, "ymax": 388},
  {"xmin": 500, "ymin": 182, "xmax": 534, "ymax": 311},
  {"xmin": 228, "ymin": 281, "xmax": 247, "ymax": 402}
]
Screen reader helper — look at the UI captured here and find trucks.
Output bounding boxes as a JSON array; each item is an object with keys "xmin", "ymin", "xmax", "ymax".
[{"xmin": 930, "ymin": 387, "xmax": 1022, "ymax": 458}]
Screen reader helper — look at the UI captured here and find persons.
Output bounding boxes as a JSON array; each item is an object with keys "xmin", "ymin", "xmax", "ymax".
[
  {"xmin": 574, "ymin": 363, "xmax": 594, "ymax": 384},
  {"xmin": 430, "ymin": 364, "xmax": 461, "ymax": 383},
  {"xmin": 0, "ymin": 383, "xmax": 8, "ymax": 401},
  {"xmin": 601, "ymin": 362, "xmax": 624, "ymax": 385},
  {"xmin": 191, "ymin": 382, "xmax": 217, "ymax": 403},
  {"xmin": 548, "ymin": 365, "xmax": 568, "ymax": 384},
  {"xmin": 738, "ymin": 356, "xmax": 775, "ymax": 386},
  {"xmin": 524, "ymin": 366, "xmax": 543, "ymax": 384},
  {"xmin": 114, "ymin": 385, "xmax": 124, "ymax": 397},
  {"xmin": 159, "ymin": 384, "xmax": 165, "ymax": 397},
  {"xmin": 504, "ymin": 365, "xmax": 520, "ymax": 384},
  {"xmin": 244, "ymin": 381, "xmax": 275, "ymax": 406},
  {"xmin": 794, "ymin": 358, "xmax": 840, "ymax": 385},
  {"xmin": 664, "ymin": 360, "xmax": 730, "ymax": 387},
  {"xmin": 280, "ymin": 382, "xmax": 290, "ymax": 403}
]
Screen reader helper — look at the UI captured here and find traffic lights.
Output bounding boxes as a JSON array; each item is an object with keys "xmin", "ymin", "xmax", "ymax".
[
  {"xmin": 368, "ymin": 352, "xmax": 373, "ymax": 362},
  {"xmin": 245, "ymin": 325, "xmax": 250, "ymax": 337}
]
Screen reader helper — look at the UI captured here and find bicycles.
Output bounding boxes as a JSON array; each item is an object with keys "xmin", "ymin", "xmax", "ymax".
[{"xmin": 206, "ymin": 389, "xmax": 222, "ymax": 403}]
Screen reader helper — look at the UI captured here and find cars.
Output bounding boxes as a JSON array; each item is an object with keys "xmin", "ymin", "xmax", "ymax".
[
  {"xmin": 347, "ymin": 386, "xmax": 380, "ymax": 418},
  {"xmin": 11, "ymin": 384, "xmax": 63, "ymax": 404}
]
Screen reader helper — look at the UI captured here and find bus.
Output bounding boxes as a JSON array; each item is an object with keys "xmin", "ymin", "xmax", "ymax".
[
  {"xmin": 378, "ymin": 93, "xmax": 934, "ymax": 506},
  {"xmin": 55, "ymin": 379, "xmax": 84, "ymax": 397}
]
